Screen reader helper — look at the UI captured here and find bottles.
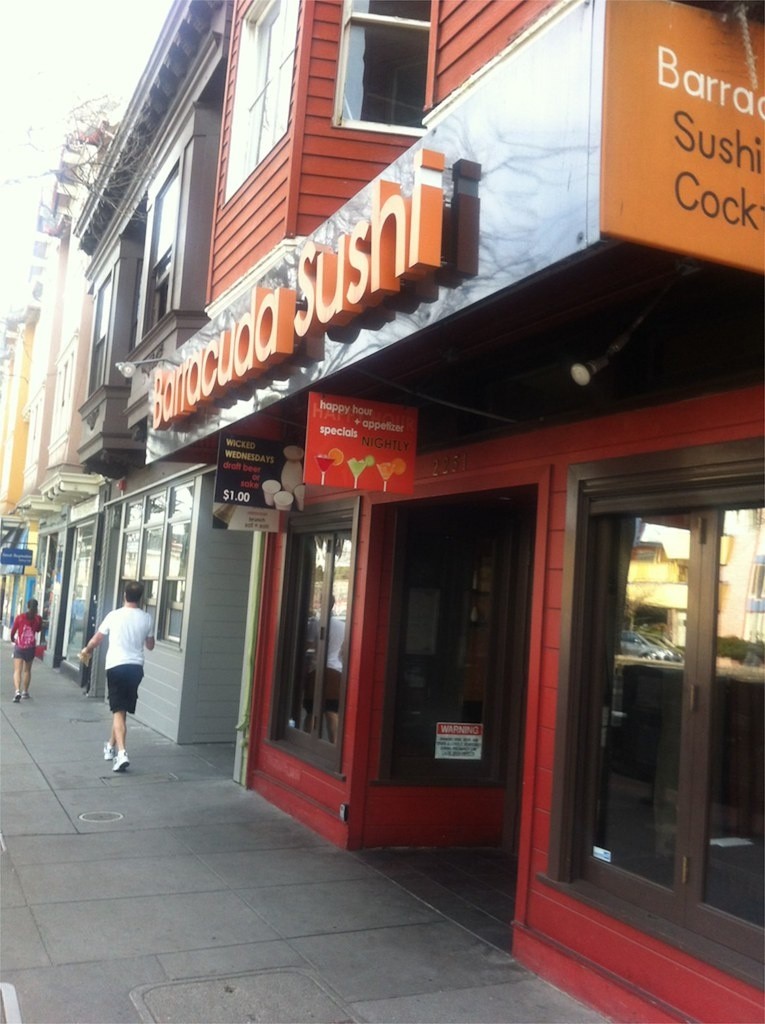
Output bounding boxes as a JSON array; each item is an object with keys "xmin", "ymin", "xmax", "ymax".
[{"xmin": 281, "ymin": 446, "xmax": 303, "ymax": 492}]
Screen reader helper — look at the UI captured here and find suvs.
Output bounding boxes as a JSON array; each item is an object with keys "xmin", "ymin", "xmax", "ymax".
[{"xmin": 619, "ymin": 630, "xmax": 686, "ymax": 666}]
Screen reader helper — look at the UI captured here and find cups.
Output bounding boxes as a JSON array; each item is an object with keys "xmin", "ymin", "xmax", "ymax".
[
  {"xmin": 262, "ymin": 480, "xmax": 281, "ymax": 506},
  {"xmin": 294, "ymin": 485, "xmax": 305, "ymax": 511},
  {"xmin": 274, "ymin": 491, "xmax": 294, "ymax": 511}
]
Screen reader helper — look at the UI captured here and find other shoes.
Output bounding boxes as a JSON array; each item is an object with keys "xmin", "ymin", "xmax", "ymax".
[
  {"xmin": 22, "ymin": 692, "xmax": 29, "ymax": 698},
  {"xmin": 12, "ymin": 690, "xmax": 21, "ymax": 702}
]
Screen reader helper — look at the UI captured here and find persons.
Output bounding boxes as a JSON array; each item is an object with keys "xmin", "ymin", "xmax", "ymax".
[
  {"xmin": 11, "ymin": 599, "xmax": 42, "ymax": 703},
  {"xmin": 68, "ymin": 592, "xmax": 77, "ymax": 644},
  {"xmin": 303, "ymin": 592, "xmax": 346, "ymax": 744},
  {"xmin": 82, "ymin": 583, "xmax": 155, "ymax": 772}
]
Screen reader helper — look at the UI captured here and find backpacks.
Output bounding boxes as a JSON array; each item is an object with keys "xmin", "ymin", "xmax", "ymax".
[{"xmin": 17, "ymin": 616, "xmax": 36, "ymax": 649}]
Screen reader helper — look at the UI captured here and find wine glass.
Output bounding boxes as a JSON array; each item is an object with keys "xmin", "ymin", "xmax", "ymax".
[
  {"xmin": 315, "ymin": 456, "xmax": 335, "ymax": 486},
  {"xmin": 348, "ymin": 462, "xmax": 369, "ymax": 489},
  {"xmin": 377, "ymin": 463, "xmax": 395, "ymax": 492}
]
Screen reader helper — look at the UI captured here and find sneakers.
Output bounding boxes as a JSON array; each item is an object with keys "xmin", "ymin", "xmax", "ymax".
[
  {"xmin": 103, "ymin": 744, "xmax": 116, "ymax": 760},
  {"xmin": 112, "ymin": 750, "xmax": 130, "ymax": 772}
]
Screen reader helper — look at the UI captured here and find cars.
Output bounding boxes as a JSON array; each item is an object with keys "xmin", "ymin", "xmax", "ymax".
[{"xmin": 70, "ymin": 597, "xmax": 86, "ymax": 641}]
